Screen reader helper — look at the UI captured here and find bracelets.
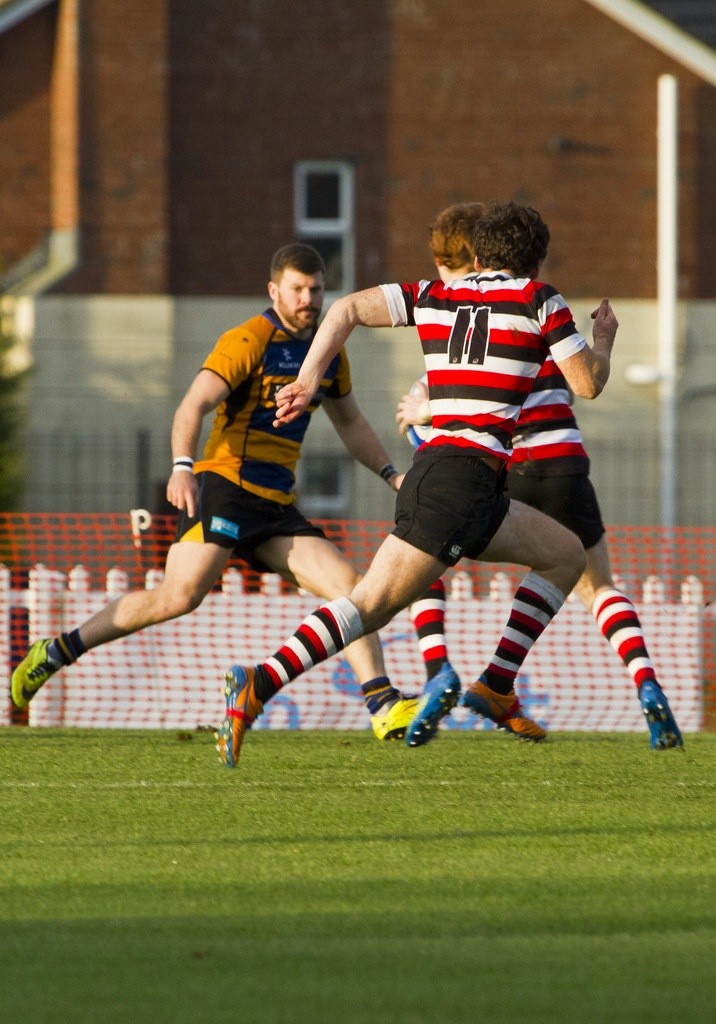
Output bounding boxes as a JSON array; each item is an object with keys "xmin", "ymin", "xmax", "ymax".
[
  {"xmin": 380, "ymin": 464, "xmax": 398, "ymax": 481},
  {"xmin": 170, "ymin": 458, "xmax": 193, "ymax": 473}
]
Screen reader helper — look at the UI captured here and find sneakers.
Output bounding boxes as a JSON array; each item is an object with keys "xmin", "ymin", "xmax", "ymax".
[
  {"xmin": 370, "ymin": 693, "xmax": 421, "ymax": 741},
  {"xmin": 640, "ymin": 681, "xmax": 683, "ymax": 750},
  {"xmin": 214, "ymin": 666, "xmax": 264, "ymax": 769},
  {"xmin": 463, "ymin": 674, "xmax": 547, "ymax": 744},
  {"xmin": 11, "ymin": 638, "xmax": 59, "ymax": 709},
  {"xmin": 405, "ymin": 662, "xmax": 462, "ymax": 748}
]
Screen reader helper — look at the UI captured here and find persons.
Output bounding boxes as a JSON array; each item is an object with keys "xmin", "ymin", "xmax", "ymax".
[
  {"xmin": 209, "ymin": 200, "xmax": 621, "ymax": 770},
  {"xmin": 13, "ymin": 243, "xmax": 460, "ymax": 745},
  {"xmin": 395, "ymin": 206, "xmax": 683, "ymax": 752}
]
